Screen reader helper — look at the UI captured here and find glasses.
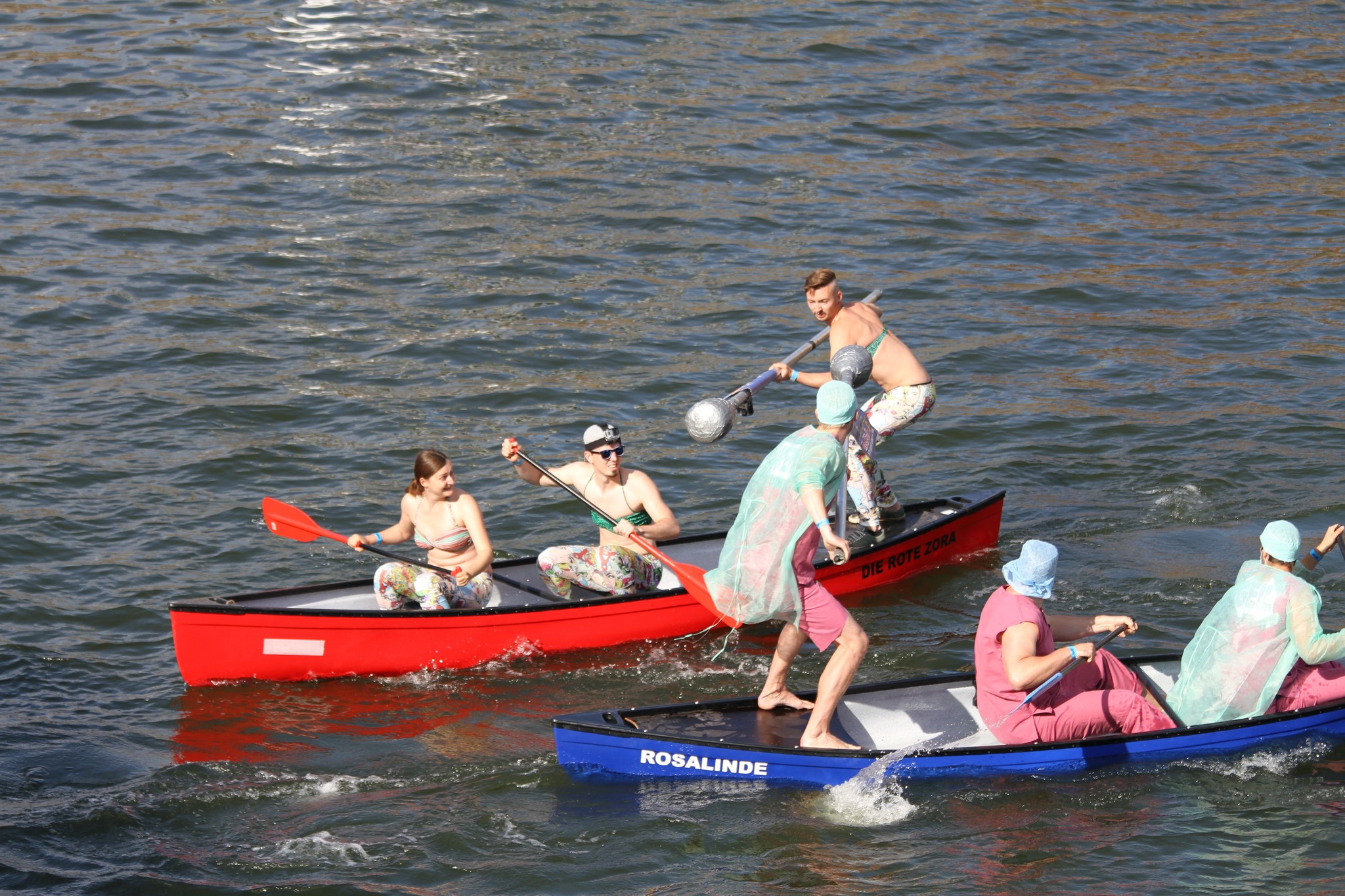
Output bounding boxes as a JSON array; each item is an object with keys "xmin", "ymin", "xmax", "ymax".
[{"xmin": 590, "ymin": 444, "xmax": 625, "ymax": 460}]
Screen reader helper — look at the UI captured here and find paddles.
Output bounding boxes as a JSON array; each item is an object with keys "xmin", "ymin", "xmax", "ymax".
[
  {"xmin": 262, "ymin": 495, "xmax": 462, "ymax": 576},
  {"xmin": 509, "ymin": 437, "xmax": 747, "ymax": 627},
  {"xmin": 1009, "ymin": 616, "xmax": 1142, "ymax": 718}
]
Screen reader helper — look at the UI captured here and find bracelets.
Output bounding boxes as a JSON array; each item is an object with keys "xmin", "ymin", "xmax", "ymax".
[
  {"xmin": 1308, "ymin": 548, "xmax": 1323, "ymax": 561},
  {"xmin": 816, "ymin": 519, "xmax": 830, "ymax": 530},
  {"xmin": 789, "ymin": 370, "xmax": 798, "ymax": 382},
  {"xmin": 514, "ymin": 458, "xmax": 524, "ymax": 465},
  {"xmin": 1068, "ymin": 644, "xmax": 1078, "ymax": 660}
]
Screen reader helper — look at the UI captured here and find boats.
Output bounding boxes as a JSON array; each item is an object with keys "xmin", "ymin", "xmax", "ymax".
[
  {"xmin": 553, "ymin": 649, "xmax": 1345, "ymax": 787},
  {"xmin": 168, "ymin": 488, "xmax": 1006, "ymax": 687}
]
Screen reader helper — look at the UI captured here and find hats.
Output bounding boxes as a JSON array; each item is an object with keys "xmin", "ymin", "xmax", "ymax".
[
  {"xmin": 1002, "ymin": 539, "xmax": 1057, "ymax": 598},
  {"xmin": 1259, "ymin": 520, "xmax": 1302, "ymax": 562},
  {"xmin": 816, "ymin": 379, "xmax": 858, "ymax": 425},
  {"xmin": 582, "ymin": 423, "xmax": 621, "ymax": 450}
]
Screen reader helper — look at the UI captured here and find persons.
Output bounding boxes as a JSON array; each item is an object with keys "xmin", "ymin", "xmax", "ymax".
[
  {"xmin": 501, "ymin": 423, "xmax": 680, "ymax": 601},
  {"xmin": 373, "ymin": 533, "xmax": 382, "ymax": 546},
  {"xmin": 348, "ymin": 449, "xmax": 493, "ymax": 612},
  {"xmin": 768, "ymin": 266, "xmax": 936, "ymax": 550},
  {"xmin": 748, "ymin": 380, "xmax": 869, "ymax": 751},
  {"xmin": 975, "ymin": 538, "xmax": 1177, "ymax": 743},
  {"xmin": 1223, "ymin": 519, "xmax": 1344, "ymax": 714}
]
